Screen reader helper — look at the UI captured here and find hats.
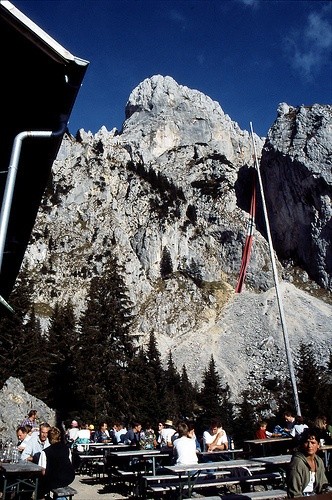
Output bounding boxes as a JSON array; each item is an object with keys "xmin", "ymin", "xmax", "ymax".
[
  {"xmin": 89, "ymin": 425, "xmax": 94, "ymax": 429},
  {"xmin": 71, "ymin": 420, "xmax": 78, "ymax": 426},
  {"xmin": 165, "ymin": 420, "xmax": 172, "ymax": 426}
]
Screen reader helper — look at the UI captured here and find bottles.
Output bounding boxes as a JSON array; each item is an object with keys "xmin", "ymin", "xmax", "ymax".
[
  {"xmin": 230, "ymin": 437, "xmax": 235, "ymax": 450},
  {"xmin": 0, "ymin": 439, "xmax": 20, "ymax": 464}
]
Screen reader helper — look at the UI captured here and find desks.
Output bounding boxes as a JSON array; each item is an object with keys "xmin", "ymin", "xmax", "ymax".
[
  {"xmin": 243, "ymin": 437, "xmax": 293, "ymax": 444},
  {"xmin": 0, "ymin": 453, "xmax": 45, "ymax": 500},
  {"xmin": 90, "ymin": 444, "xmax": 161, "ymax": 457},
  {"xmin": 143, "ymin": 450, "xmax": 294, "ymax": 500}
]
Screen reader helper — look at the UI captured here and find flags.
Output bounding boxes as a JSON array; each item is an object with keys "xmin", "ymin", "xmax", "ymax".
[{"xmin": 235, "ymin": 186, "xmax": 257, "ymax": 293}]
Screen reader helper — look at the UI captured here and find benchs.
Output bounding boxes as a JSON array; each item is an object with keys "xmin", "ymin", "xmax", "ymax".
[
  {"xmin": 118, "ymin": 467, "xmax": 287, "ymax": 500},
  {"xmin": 51, "ymin": 486, "xmax": 78, "ymax": 500},
  {"xmin": 77, "ymin": 455, "xmax": 104, "ymax": 476}
]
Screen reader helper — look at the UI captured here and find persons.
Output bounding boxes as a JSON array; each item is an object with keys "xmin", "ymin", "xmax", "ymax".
[
  {"xmin": 300, "ymin": 416, "xmax": 332, "ymax": 445},
  {"xmin": 12, "ymin": 410, "xmax": 201, "ymax": 500},
  {"xmin": 286, "ymin": 427, "xmax": 332, "ymax": 500},
  {"xmin": 289, "ymin": 416, "xmax": 309, "ymax": 445},
  {"xmin": 255, "ymin": 421, "xmax": 282, "ymax": 439},
  {"xmin": 284, "ymin": 412, "xmax": 296, "ymax": 432},
  {"xmin": 203, "ymin": 418, "xmax": 230, "ymax": 479},
  {"xmin": 24, "ymin": 426, "xmax": 75, "ymax": 500},
  {"xmin": 172, "ymin": 423, "xmax": 199, "ymax": 465}
]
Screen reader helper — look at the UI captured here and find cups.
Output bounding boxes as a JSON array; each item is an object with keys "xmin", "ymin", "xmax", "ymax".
[
  {"xmin": 22, "ymin": 457, "xmax": 28, "ymax": 465},
  {"xmin": 320, "ymin": 438, "xmax": 324, "ymax": 447}
]
